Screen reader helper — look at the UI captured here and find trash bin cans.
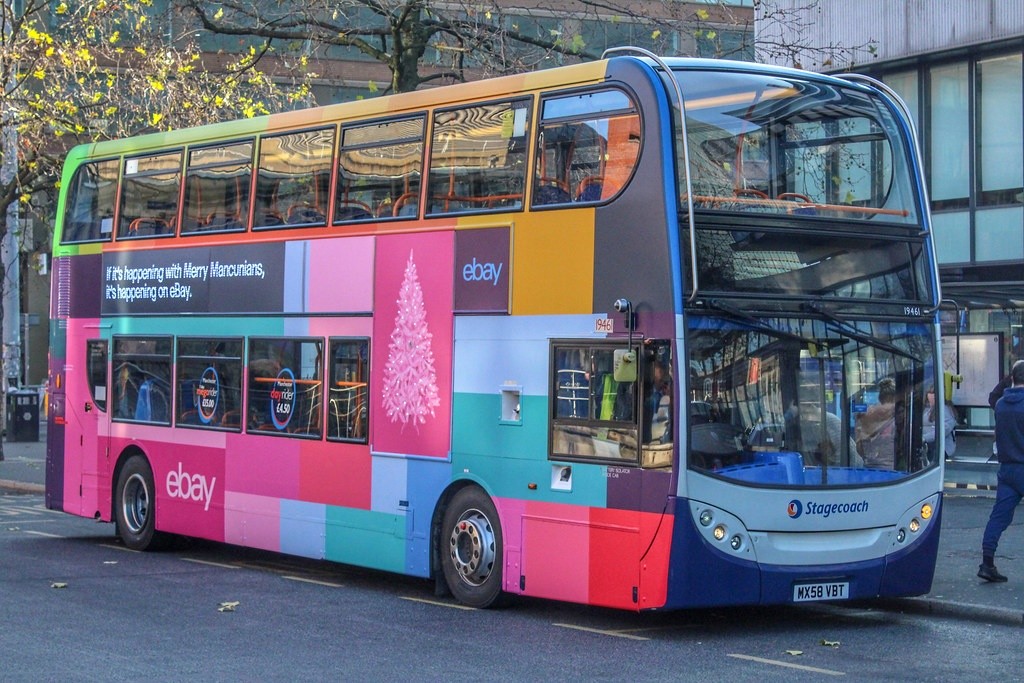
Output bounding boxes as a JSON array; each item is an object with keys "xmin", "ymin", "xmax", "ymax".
[{"xmin": 5, "ymin": 390, "xmax": 40, "ymax": 443}]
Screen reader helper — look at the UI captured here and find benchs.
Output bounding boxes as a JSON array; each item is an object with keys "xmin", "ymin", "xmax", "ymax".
[{"xmin": 946, "ymin": 455, "xmax": 1001, "ymax": 465}]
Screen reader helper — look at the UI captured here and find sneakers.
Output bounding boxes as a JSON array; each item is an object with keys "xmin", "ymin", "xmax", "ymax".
[{"xmin": 977, "ymin": 563, "xmax": 1008, "ymax": 582}]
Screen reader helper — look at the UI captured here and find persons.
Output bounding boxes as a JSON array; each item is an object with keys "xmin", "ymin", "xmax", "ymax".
[
  {"xmin": 784, "ymin": 370, "xmax": 1009, "ymax": 468},
  {"xmin": 977, "ymin": 361, "xmax": 1024, "ymax": 582},
  {"xmin": 613, "ymin": 354, "xmax": 691, "ymax": 449}
]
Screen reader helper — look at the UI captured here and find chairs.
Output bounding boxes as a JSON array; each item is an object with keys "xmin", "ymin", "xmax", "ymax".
[
  {"xmin": 99, "ymin": 355, "xmax": 370, "ymax": 443},
  {"xmin": 125, "ymin": 174, "xmax": 815, "ymax": 237}
]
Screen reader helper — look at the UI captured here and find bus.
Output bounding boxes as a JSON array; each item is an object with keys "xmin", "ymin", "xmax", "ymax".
[{"xmin": 45, "ymin": 44, "xmax": 963, "ymax": 617}]
